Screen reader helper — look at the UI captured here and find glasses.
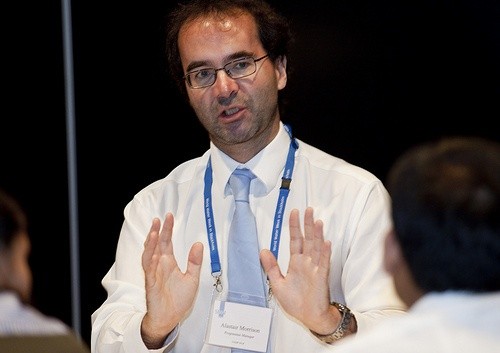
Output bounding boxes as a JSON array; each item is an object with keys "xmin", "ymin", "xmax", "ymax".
[{"xmin": 183, "ymin": 53, "xmax": 271, "ymax": 89}]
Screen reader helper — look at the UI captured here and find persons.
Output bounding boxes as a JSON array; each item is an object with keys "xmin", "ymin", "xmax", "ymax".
[
  {"xmin": 93, "ymin": 0, "xmax": 409, "ymax": 353},
  {"xmin": 326, "ymin": 138, "xmax": 500, "ymax": 353},
  {"xmin": 0, "ymin": 198, "xmax": 88, "ymax": 353}
]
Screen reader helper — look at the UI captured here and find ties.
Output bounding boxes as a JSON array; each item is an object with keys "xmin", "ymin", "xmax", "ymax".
[{"xmin": 227, "ymin": 169, "xmax": 271, "ymax": 352}]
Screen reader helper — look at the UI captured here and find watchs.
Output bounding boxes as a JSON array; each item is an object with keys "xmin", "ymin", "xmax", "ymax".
[{"xmin": 310, "ymin": 302, "xmax": 351, "ymax": 345}]
